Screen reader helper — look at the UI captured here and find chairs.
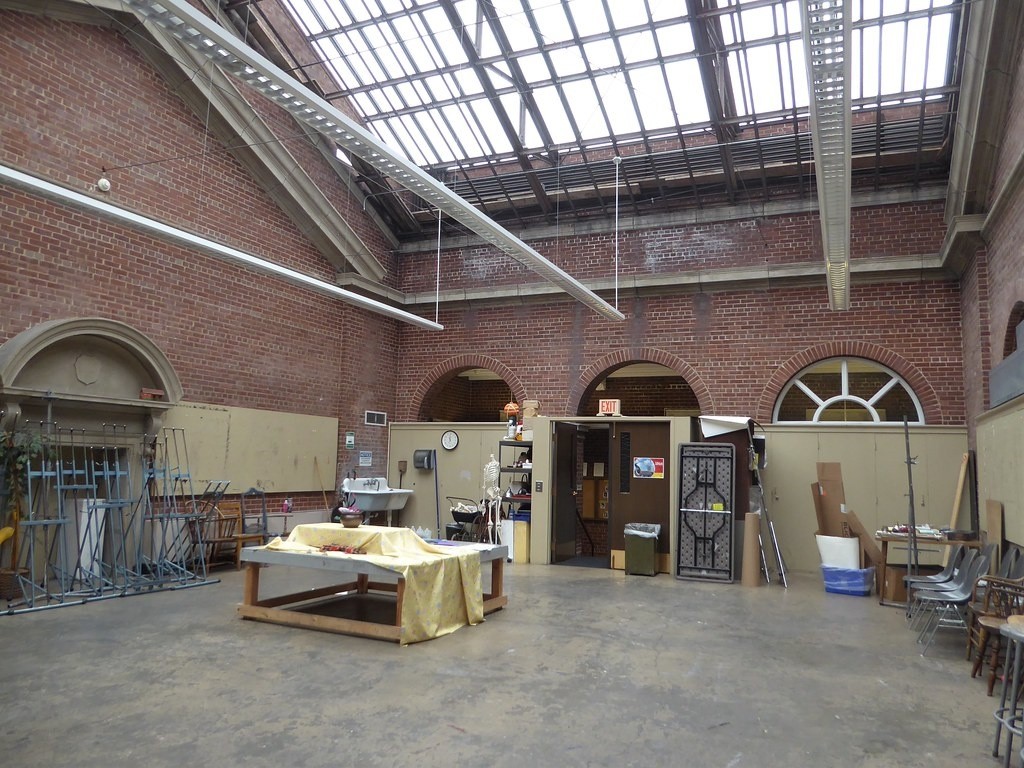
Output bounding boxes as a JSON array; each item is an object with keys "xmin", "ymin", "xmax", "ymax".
[
  {"xmin": 902, "ymin": 543, "xmax": 1020, "ymax": 657},
  {"xmin": 185, "ymin": 500, "xmax": 237, "ymax": 576},
  {"xmin": 966, "ymin": 574, "xmax": 1024, "ymax": 665},
  {"xmin": 214, "ymin": 502, "xmax": 264, "ymax": 571}
]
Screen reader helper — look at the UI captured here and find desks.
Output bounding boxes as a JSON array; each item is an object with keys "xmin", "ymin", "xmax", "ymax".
[
  {"xmin": 239, "ymin": 523, "xmax": 510, "ymax": 648},
  {"xmin": 875, "ymin": 525, "xmax": 977, "ymax": 605}
]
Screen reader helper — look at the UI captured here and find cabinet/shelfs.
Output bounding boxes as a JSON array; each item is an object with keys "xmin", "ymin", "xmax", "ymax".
[{"xmin": 498, "ymin": 440, "xmax": 532, "ymax": 565}]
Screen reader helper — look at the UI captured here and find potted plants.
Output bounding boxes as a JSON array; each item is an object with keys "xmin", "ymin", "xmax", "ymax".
[{"xmin": 0, "ymin": 422, "xmax": 63, "ymax": 600}]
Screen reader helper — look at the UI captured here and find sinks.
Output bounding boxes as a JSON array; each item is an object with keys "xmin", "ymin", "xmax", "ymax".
[{"xmin": 343, "ymin": 478, "xmax": 414, "ymax": 511}]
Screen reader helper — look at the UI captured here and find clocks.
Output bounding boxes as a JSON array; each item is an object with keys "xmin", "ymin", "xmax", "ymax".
[{"xmin": 441, "ymin": 430, "xmax": 459, "ymax": 451}]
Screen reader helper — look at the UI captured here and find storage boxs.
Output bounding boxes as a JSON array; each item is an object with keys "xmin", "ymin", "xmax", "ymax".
[{"xmin": 819, "ymin": 564, "xmax": 876, "ymax": 596}]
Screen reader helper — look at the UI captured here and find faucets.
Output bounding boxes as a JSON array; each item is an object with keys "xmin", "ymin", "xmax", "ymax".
[{"xmin": 363, "ymin": 478, "xmax": 380, "ymax": 491}]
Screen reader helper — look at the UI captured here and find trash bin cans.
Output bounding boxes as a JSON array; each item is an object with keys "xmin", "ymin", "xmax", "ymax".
[
  {"xmin": 623, "ymin": 522, "xmax": 662, "ymax": 577},
  {"xmin": 446, "ymin": 522, "xmax": 464, "ymax": 540}
]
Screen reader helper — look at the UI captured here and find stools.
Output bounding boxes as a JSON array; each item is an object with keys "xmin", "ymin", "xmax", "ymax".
[
  {"xmin": 971, "ymin": 616, "xmax": 1007, "ymax": 697},
  {"xmin": 992, "ymin": 624, "xmax": 1024, "ymax": 768}
]
